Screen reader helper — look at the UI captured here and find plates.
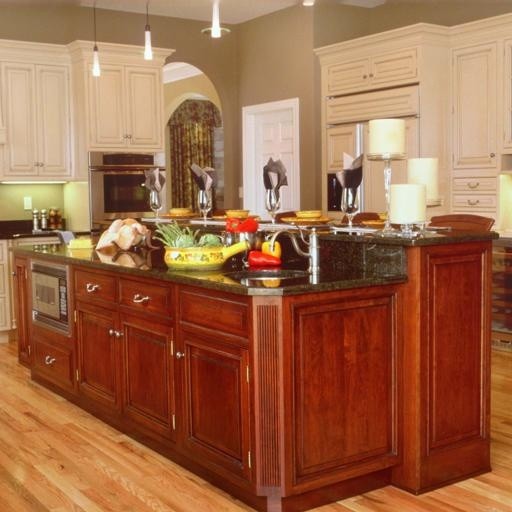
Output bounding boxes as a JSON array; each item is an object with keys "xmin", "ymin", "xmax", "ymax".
[
  {"xmin": 281, "ymin": 215, "xmax": 335, "ymax": 225},
  {"xmin": 213, "ymin": 214, "xmax": 260, "ymax": 221},
  {"xmin": 160, "ymin": 213, "xmax": 201, "ymax": 218},
  {"xmin": 363, "ymin": 218, "xmax": 387, "ymax": 230}
]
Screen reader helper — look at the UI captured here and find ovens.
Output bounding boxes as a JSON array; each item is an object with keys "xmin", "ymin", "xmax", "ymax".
[{"xmin": 89, "ymin": 169, "xmax": 167, "ymax": 220}]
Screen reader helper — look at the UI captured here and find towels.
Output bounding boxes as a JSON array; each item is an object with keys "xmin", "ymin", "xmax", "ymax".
[
  {"xmin": 262, "ymin": 157, "xmax": 288, "ymax": 210},
  {"xmin": 143, "ymin": 164, "xmax": 215, "ymax": 208}
]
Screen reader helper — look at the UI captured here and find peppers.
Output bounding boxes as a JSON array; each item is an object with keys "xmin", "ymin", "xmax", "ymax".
[
  {"xmin": 247, "ymin": 250, "xmax": 284, "ymax": 267},
  {"xmin": 260, "ymin": 241, "xmax": 282, "ymax": 258}
]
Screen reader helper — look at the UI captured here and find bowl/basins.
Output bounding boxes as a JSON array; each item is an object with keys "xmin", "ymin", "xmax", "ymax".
[
  {"xmin": 296, "ymin": 209, "xmax": 322, "ymax": 220},
  {"xmin": 168, "ymin": 206, "xmax": 192, "ymax": 214},
  {"xmin": 224, "ymin": 210, "xmax": 250, "ymax": 218},
  {"xmin": 377, "ymin": 211, "xmax": 389, "ymax": 221}
]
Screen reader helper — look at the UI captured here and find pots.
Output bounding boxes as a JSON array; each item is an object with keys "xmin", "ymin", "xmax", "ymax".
[{"xmin": 165, "ymin": 241, "xmax": 250, "ymax": 270}]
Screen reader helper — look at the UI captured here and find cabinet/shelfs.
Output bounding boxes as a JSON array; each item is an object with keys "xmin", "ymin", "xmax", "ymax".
[
  {"xmin": 0, "ymin": 38, "xmax": 177, "ymax": 185},
  {"xmin": 175, "ymin": 282, "xmax": 406, "ymax": 512},
  {"xmin": 11, "ymin": 255, "xmax": 33, "ymax": 368},
  {"xmin": 71, "ymin": 263, "xmax": 179, "ymax": 465}
]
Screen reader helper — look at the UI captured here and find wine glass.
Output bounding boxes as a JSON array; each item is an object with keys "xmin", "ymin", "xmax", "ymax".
[
  {"xmin": 147, "ymin": 189, "xmax": 164, "ymax": 220},
  {"xmin": 264, "ymin": 189, "xmax": 282, "ymax": 229},
  {"xmin": 339, "ymin": 187, "xmax": 361, "ymax": 232},
  {"xmin": 195, "ymin": 188, "xmax": 214, "ymax": 223}
]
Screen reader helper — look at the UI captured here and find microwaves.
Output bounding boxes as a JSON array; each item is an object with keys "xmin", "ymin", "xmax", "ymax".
[{"xmin": 30, "ymin": 262, "xmax": 69, "ymax": 334}]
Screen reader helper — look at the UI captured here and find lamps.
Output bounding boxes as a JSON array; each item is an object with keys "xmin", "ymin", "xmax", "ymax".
[{"xmin": 93, "ymin": 1, "xmax": 154, "ymax": 80}]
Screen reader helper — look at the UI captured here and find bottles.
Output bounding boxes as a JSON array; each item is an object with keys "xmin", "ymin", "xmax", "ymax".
[
  {"xmin": 32, "ymin": 206, "xmax": 47, "ymax": 230},
  {"xmin": 49, "ymin": 208, "xmax": 66, "ymax": 229}
]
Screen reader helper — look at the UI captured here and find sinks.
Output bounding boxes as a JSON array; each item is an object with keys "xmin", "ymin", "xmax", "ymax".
[{"xmin": 224, "ymin": 269, "xmax": 308, "ymax": 281}]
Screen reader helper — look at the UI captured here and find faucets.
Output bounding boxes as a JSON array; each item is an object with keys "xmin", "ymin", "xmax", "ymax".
[{"xmin": 269, "ymin": 221, "xmax": 320, "ymax": 285}]
